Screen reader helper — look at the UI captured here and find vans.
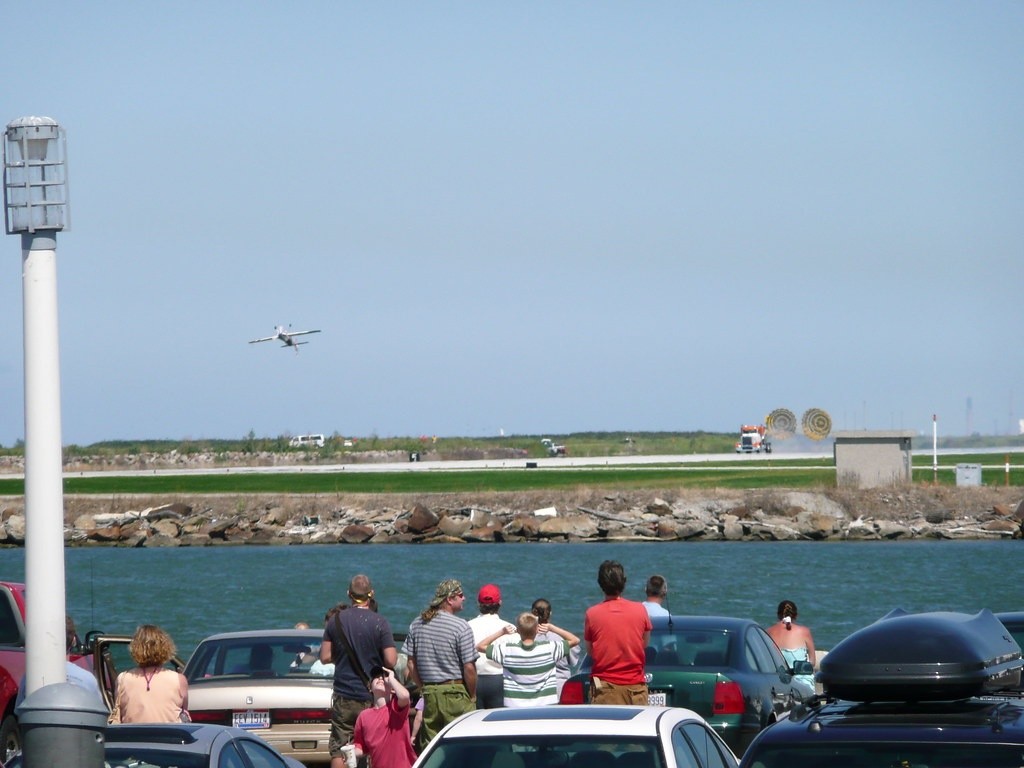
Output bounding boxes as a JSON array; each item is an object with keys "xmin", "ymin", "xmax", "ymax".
[{"xmin": 287, "ymin": 435, "xmax": 325, "ymax": 449}]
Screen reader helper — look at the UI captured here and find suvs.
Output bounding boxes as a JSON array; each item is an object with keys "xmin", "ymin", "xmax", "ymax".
[{"xmin": 736, "ymin": 605, "xmax": 1023, "ymax": 768}]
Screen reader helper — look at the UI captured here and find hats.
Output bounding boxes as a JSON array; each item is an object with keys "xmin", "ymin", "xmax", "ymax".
[
  {"xmin": 430, "ymin": 578, "xmax": 460, "ymax": 606},
  {"xmin": 369, "ymin": 666, "xmax": 389, "ymax": 680},
  {"xmin": 478, "ymin": 584, "xmax": 501, "ymax": 603}
]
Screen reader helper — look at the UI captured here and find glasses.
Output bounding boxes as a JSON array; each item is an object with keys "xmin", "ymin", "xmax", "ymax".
[{"xmin": 454, "ymin": 591, "xmax": 463, "ymax": 598}]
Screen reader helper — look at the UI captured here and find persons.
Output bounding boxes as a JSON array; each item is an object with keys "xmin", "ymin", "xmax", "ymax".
[
  {"xmin": 14, "ymin": 615, "xmax": 104, "ymax": 716},
  {"xmin": 475, "ymin": 611, "xmax": 581, "ymax": 708},
  {"xmin": 108, "ymin": 624, "xmax": 189, "ymax": 724},
  {"xmin": 641, "ymin": 574, "xmax": 678, "ymax": 650},
  {"xmin": 292, "ymin": 599, "xmax": 379, "ymax": 675},
  {"xmin": 584, "ymin": 560, "xmax": 653, "ymax": 754},
  {"xmin": 533, "ymin": 598, "xmax": 580, "ymax": 700},
  {"xmin": 431, "ymin": 435, "xmax": 437, "ymax": 443},
  {"xmin": 342, "ymin": 664, "xmax": 419, "ymax": 768},
  {"xmin": 466, "ymin": 582, "xmax": 521, "ymax": 708},
  {"xmin": 400, "ymin": 579, "xmax": 482, "ymax": 752},
  {"xmin": 765, "ymin": 600, "xmax": 817, "ymax": 694},
  {"xmin": 320, "ymin": 575, "xmax": 398, "ymax": 768}
]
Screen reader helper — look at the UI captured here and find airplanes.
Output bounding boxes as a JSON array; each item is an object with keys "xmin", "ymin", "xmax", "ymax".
[{"xmin": 248, "ymin": 323, "xmax": 326, "ymax": 358}]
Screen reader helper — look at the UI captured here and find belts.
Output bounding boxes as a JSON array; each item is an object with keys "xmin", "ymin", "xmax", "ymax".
[{"xmin": 422, "ymin": 678, "xmax": 464, "ymax": 685}]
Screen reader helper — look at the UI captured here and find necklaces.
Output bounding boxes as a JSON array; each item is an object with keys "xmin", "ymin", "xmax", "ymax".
[{"xmin": 142, "ymin": 663, "xmax": 157, "ymax": 691}]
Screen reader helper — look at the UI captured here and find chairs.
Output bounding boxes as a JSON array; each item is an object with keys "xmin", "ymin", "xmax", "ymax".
[
  {"xmin": 644, "ymin": 647, "xmax": 657, "ymax": 665},
  {"xmin": 615, "ymin": 752, "xmax": 651, "ymax": 768},
  {"xmin": 695, "ymin": 651, "xmax": 728, "ymax": 665},
  {"xmin": 568, "ymin": 750, "xmax": 615, "ymax": 768}
]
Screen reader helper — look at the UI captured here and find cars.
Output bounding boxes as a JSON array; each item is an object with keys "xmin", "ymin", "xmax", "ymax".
[
  {"xmin": 89, "ymin": 628, "xmax": 346, "ymax": 768},
  {"xmin": 0, "ymin": 581, "xmax": 120, "ymax": 725},
  {"xmin": 409, "ymin": 704, "xmax": 763, "ymax": 767},
  {"xmin": 541, "ymin": 439, "xmax": 567, "ymax": 456},
  {"xmin": 557, "ymin": 613, "xmax": 813, "ymax": 764},
  {"xmin": 1, "ymin": 721, "xmax": 307, "ymax": 767}
]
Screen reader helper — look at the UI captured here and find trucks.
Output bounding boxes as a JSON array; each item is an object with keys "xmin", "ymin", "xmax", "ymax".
[{"xmin": 735, "ymin": 425, "xmax": 773, "ymax": 455}]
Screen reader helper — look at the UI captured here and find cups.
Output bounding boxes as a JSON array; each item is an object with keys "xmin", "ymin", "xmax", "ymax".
[{"xmin": 339, "ymin": 745, "xmax": 356, "ymax": 768}]
